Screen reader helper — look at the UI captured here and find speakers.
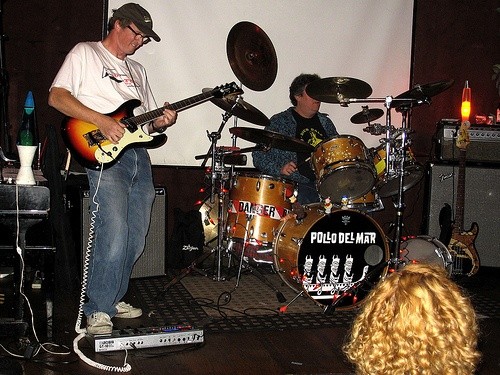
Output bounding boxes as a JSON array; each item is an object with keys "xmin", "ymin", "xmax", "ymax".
[
  {"xmin": 66, "ymin": 173, "xmax": 167, "ymax": 282},
  {"xmin": 434, "ymin": 123, "xmax": 500, "ymax": 165},
  {"xmin": 425, "ymin": 160, "xmax": 500, "ymax": 275}
]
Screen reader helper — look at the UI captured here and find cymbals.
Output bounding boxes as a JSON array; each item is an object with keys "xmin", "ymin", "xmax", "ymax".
[
  {"xmin": 304, "ymin": 77, "xmax": 373, "ymax": 104},
  {"xmin": 201, "ymin": 87, "xmax": 271, "ymax": 126},
  {"xmin": 350, "ymin": 108, "xmax": 384, "ymax": 124},
  {"xmin": 228, "ymin": 127, "xmax": 318, "ymax": 153},
  {"xmin": 384, "ymin": 78, "xmax": 455, "ymax": 108},
  {"xmin": 226, "ymin": 21, "xmax": 277, "ymax": 92}
]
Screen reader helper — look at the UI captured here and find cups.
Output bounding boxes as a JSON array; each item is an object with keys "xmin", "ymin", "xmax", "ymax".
[{"xmin": 475, "ymin": 114, "xmax": 496, "ymax": 126}]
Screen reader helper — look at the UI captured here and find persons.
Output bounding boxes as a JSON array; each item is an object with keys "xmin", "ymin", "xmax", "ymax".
[
  {"xmin": 252, "ymin": 73, "xmax": 339, "ymax": 205},
  {"xmin": 47, "ymin": 2, "xmax": 177, "ymax": 337},
  {"xmin": 342, "ymin": 263, "xmax": 482, "ymax": 375}
]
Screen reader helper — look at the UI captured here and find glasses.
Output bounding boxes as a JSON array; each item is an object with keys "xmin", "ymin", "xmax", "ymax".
[{"xmin": 127, "ymin": 26, "xmax": 151, "ymax": 44}]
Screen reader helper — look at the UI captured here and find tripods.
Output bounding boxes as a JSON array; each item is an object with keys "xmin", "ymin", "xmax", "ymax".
[{"xmin": 156, "ymin": 93, "xmax": 426, "ymax": 317}]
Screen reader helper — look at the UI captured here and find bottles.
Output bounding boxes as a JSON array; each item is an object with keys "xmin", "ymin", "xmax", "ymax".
[{"xmin": 496, "ymin": 104, "xmax": 500, "ymax": 124}]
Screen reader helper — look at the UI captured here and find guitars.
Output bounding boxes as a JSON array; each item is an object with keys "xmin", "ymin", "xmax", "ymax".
[
  {"xmin": 439, "ymin": 121, "xmax": 481, "ymax": 279},
  {"xmin": 61, "ymin": 80, "xmax": 245, "ymax": 171}
]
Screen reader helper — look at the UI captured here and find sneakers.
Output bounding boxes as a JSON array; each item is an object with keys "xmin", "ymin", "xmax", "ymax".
[
  {"xmin": 115, "ymin": 302, "xmax": 142, "ymax": 318},
  {"xmin": 87, "ymin": 312, "xmax": 113, "ymax": 334}
]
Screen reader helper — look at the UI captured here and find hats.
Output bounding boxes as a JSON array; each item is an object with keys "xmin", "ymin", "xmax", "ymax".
[{"xmin": 118, "ymin": 3, "xmax": 160, "ymax": 42}]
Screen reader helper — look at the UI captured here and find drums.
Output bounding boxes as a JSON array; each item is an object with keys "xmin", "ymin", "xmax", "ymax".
[
  {"xmin": 272, "ymin": 201, "xmax": 390, "ymax": 311},
  {"xmin": 226, "ymin": 171, "xmax": 300, "ymax": 248},
  {"xmin": 399, "ymin": 233, "xmax": 453, "ymax": 283},
  {"xmin": 309, "ymin": 134, "xmax": 377, "ymax": 201},
  {"xmin": 373, "ymin": 138, "xmax": 425, "ymax": 199},
  {"xmin": 341, "ymin": 185, "xmax": 380, "ymax": 209}
]
